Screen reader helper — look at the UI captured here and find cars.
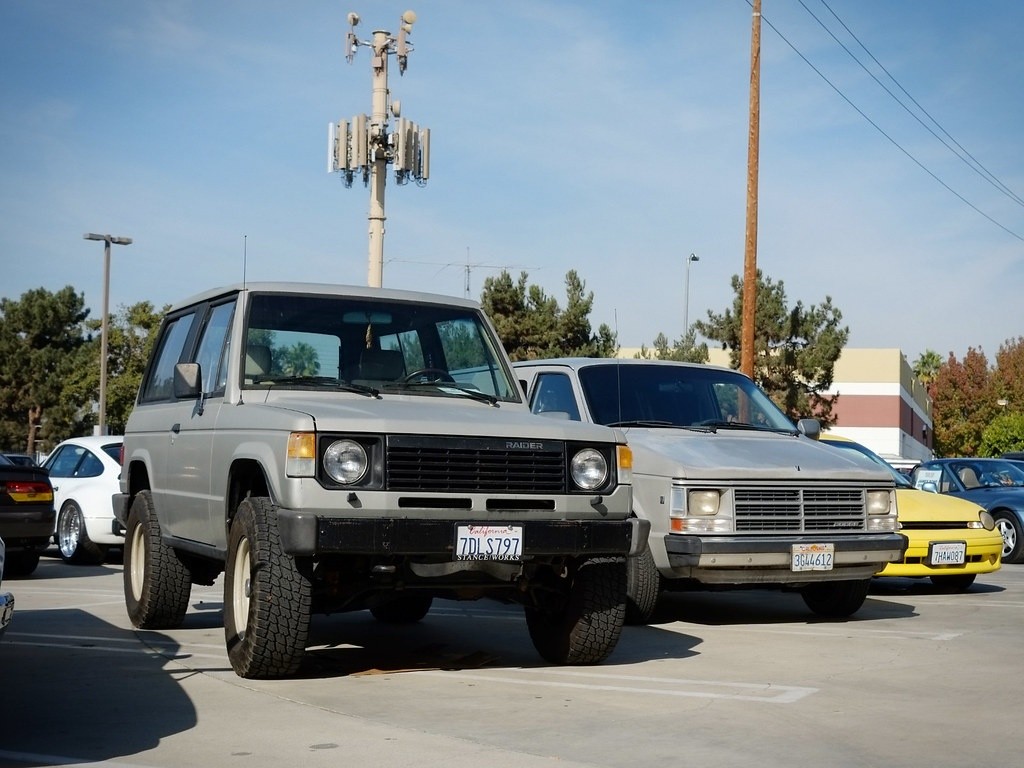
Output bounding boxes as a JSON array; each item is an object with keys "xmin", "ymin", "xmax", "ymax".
[{"xmin": 0, "ymin": 437, "xmax": 127, "ymax": 579}]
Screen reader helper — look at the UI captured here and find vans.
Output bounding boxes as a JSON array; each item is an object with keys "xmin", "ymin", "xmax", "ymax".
[{"xmin": 442, "ymin": 358, "xmax": 1024, "ymax": 626}]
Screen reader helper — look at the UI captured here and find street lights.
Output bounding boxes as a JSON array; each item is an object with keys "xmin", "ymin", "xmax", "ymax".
[
  {"xmin": 84, "ymin": 232, "xmax": 134, "ymax": 438},
  {"xmin": 685, "ymin": 252, "xmax": 697, "ymax": 342}
]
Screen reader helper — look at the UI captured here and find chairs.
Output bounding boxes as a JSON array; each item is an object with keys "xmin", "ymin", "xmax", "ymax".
[
  {"xmin": 360, "ymin": 352, "xmax": 406, "ymax": 382},
  {"xmin": 242, "ymin": 345, "xmax": 275, "ymax": 387}
]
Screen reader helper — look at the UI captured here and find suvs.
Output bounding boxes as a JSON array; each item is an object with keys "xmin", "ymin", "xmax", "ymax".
[{"xmin": 113, "ymin": 286, "xmax": 651, "ymax": 681}]
